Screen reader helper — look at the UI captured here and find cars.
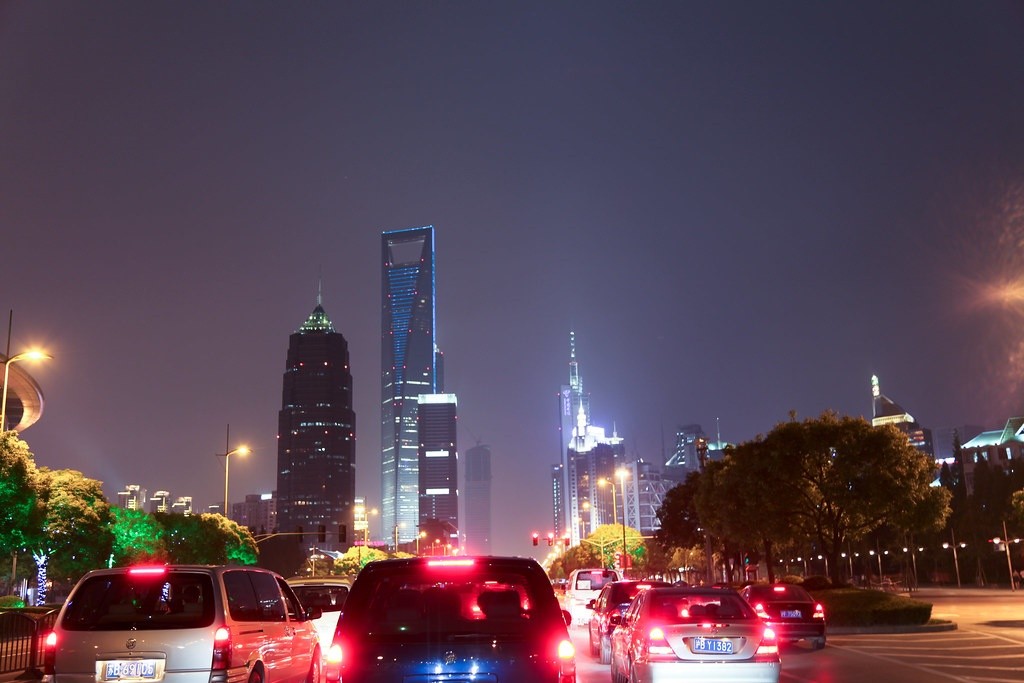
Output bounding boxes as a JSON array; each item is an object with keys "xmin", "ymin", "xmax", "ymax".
[
  {"xmin": 587, "ymin": 581, "xmax": 672, "ymax": 662},
  {"xmin": 740, "ymin": 583, "xmax": 828, "ymax": 650},
  {"xmin": 326, "ymin": 555, "xmax": 578, "ymax": 683},
  {"xmin": 609, "ymin": 586, "xmax": 784, "ymax": 683},
  {"xmin": 286, "ymin": 576, "xmax": 351, "ymax": 652}
]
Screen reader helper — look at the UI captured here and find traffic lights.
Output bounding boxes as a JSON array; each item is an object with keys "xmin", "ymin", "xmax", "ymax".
[
  {"xmin": 565, "ymin": 534, "xmax": 570, "ymax": 546},
  {"xmin": 548, "ymin": 533, "xmax": 554, "ymax": 547},
  {"xmin": 745, "ymin": 553, "xmax": 758, "ymax": 568},
  {"xmin": 532, "ymin": 533, "xmax": 537, "ymax": 546}
]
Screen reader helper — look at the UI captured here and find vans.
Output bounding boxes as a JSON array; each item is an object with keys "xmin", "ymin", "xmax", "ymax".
[
  {"xmin": 44, "ymin": 564, "xmax": 324, "ymax": 683},
  {"xmin": 560, "ymin": 570, "xmax": 625, "ymax": 626}
]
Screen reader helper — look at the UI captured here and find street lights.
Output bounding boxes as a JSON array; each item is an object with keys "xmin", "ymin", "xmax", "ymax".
[
  {"xmin": 416, "ymin": 531, "xmax": 426, "ymax": 554},
  {"xmin": 616, "ymin": 467, "xmax": 628, "ymax": 530},
  {"xmin": 943, "ymin": 543, "xmax": 966, "ymax": 587},
  {"xmin": 994, "ymin": 537, "xmax": 1020, "ymax": 592},
  {"xmin": 598, "ymin": 478, "xmax": 617, "ymax": 524},
  {"xmin": 224, "ymin": 445, "xmax": 254, "ymax": 515},
  {"xmin": 841, "ymin": 552, "xmax": 859, "ymax": 576},
  {"xmin": 455, "ymin": 546, "xmax": 464, "ymax": 555},
  {"xmin": 780, "ymin": 554, "xmax": 830, "ymax": 577},
  {"xmin": 903, "ymin": 547, "xmax": 923, "ymax": 586},
  {"xmin": 0, "ymin": 351, "xmax": 55, "ymax": 435},
  {"xmin": 364, "ymin": 509, "xmax": 378, "ymax": 544},
  {"xmin": 583, "ymin": 502, "xmax": 609, "ymax": 524},
  {"xmin": 355, "ymin": 505, "xmax": 364, "ymax": 568},
  {"xmin": 444, "ymin": 543, "xmax": 451, "ymax": 554},
  {"xmin": 394, "ymin": 523, "xmax": 406, "ymax": 554},
  {"xmin": 870, "ymin": 550, "xmax": 889, "ymax": 585},
  {"xmin": 432, "ymin": 539, "xmax": 440, "ymax": 555}
]
